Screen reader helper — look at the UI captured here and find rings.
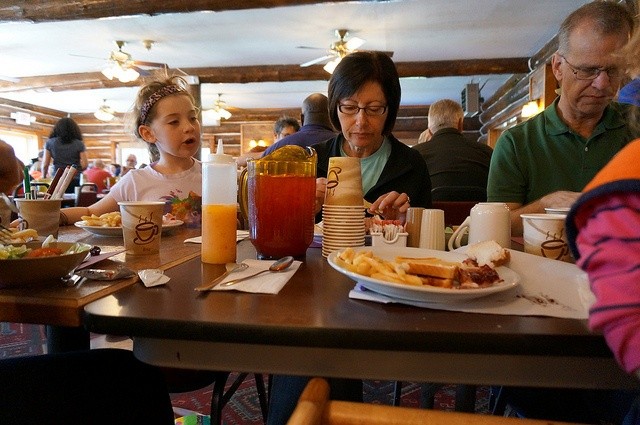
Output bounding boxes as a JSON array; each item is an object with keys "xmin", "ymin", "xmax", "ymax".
[{"xmin": 407, "ymin": 195, "xmax": 410, "ymax": 201}]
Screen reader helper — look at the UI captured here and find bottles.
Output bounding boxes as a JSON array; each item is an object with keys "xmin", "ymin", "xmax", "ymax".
[
  {"xmin": 201, "ymin": 139, "xmax": 238, "ymax": 264},
  {"xmin": 79, "ymin": 174, "xmax": 89, "ymax": 186}
]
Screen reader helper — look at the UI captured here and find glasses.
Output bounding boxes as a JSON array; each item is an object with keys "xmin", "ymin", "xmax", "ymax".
[
  {"xmin": 558, "ymin": 55, "xmax": 630, "ymax": 80},
  {"xmin": 337, "ymin": 102, "xmax": 388, "ymax": 116},
  {"xmin": 277, "ymin": 132, "xmax": 290, "ymax": 138}
]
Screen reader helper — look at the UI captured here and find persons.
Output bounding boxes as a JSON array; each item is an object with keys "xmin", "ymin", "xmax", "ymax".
[
  {"xmin": 237, "ymin": 113, "xmax": 300, "ymax": 166},
  {"xmin": 0, "ymin": 141, "xmax": 25, "ymax": 227},
  {"xmin": 29, "ymin": 151, "xmax": 43, "ymax": 175},
  {"xmin": 307, "ymin": 50, "xmax": 430, "ymax": 223},
  {"xmin": 261, "ymin": 94, "xmax": 339, "ymax": 222},
  {"xmin": 43, "ymin": 119, "xmax": 88, "ymax": 193},
  {"xmin": 84, "ymin": 159, "xmax": 116, "ymax": 193},
  {"xmin": 118, "ymin": 155, "xmax": 136, "ymax": 178},
  {"xmin": 410, "ymin": 98, "xmax": 492, "ymax": 203},
  {"xmin": 613, "ymin": 24, "xmax": 640, "ymax": 107},
  {"xmin": 486, "ymin": 0, "xmax": 639, "ymax": 236},
  {"xmin": 5, "ymin": 67, "xmax": 202, "ymax": 230},
  {"xmin": 561, "ymin": 135, "xmax": 638, "ymax": 389}
]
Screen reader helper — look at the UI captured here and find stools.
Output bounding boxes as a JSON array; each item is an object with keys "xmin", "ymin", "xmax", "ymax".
[{"xmin": 164, "ymin": 368, "xmax": 268, "ymax": 425}]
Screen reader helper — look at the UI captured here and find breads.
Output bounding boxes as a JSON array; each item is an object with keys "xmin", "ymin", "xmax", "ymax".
[{"xmin": 396, "ymin": 257, "xmax": 461, "ymax": 288}]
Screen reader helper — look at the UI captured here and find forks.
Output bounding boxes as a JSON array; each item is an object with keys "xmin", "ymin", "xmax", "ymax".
[
  {"xmin": 0, "ymin": 224, "xmax": 13, "ymax": 245},
  {"xmin": 193, "ymin": 262, "xmax": 249, "ymax": 293}
]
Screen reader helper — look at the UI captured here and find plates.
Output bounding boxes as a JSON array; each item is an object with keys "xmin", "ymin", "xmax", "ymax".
[
  {"xmin": 327, "ymin": 246, "xmax": 520, "ymax": 304},
  {"xmin": 312, "ymin": 234, "xmax": 371, "ymax": 242},
  {"xmin": 74, "ymin": 220, "xmax": 184, "ymax": 237}
]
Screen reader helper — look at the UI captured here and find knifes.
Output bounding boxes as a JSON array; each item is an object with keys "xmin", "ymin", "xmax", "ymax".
[{"xmin": 0, "ymin": 188, "xmax": 19, "ymax": 213}]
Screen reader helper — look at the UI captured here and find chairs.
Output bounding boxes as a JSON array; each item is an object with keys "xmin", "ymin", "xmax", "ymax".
[
  {"xmin": 74, "ymin": 185, "xmax": 98, "ymax": 205},
  {"xmin": 431, "ymin": 185, "xmax": 488, "ymax": 224},
  {"xmin": 14, "ymin": 182, "xmax": 50, "ymax": 199}
]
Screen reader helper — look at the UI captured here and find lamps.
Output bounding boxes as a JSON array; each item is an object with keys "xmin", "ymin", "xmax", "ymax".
[
  {"xmin": 93, "ymin": 99, "xmax": 116, "ymax": 125},
  {"xmin": 324, "ymin": 40, "xmax": 364, "ymax": 73},
  {"xmin": 98, "ymin": 43, "xmax": 140, "ymax": 83},
  {"xmin": 201, "ymin": 95, "xmax": 233, "ymax": 127}
]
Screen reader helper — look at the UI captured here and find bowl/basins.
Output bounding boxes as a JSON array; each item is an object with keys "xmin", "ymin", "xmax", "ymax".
[{"xmin": 0, "ymin": 241, "xmax": 92, "ymax": 287}]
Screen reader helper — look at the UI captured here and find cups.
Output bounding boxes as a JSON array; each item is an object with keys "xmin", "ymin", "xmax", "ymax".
[
  {"xmin": 322, "ymin": 157, "xmax": 366, "ymax": 259},
  {"xmin": 520, "ymin": 213, "xmax": 572, "ymax": 262},
  {"xmin": 418, "ymin": 209, "xmax": 446, "ymax": 251},
  {"xmin": 544, "ymin": 207, "xmax": 571, "ymax": 214},
  {"xmin": 448, "ymin": 202, "xmax": 511, "ymax": 251},
  {"xmin": 14, "ymin": 197, "xmax": 64, "ymax": 240},
  {"xmin": 405, "ymin": 207, "xmax": 425, "ymax": 248},
  {"xmin": 117, "ymin": 202, "xmax": 166, "ymax": 256},
  {"xmin": 103, "ymin": 176, "xmax": 121, "ymax": 189},
  {"xmin": 247, "ymin": 145, "xmax": 316, "ymax": 261}
]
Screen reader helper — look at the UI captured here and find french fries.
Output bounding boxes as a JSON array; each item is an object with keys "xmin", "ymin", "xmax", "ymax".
[
  {"xmin": 82, "ymin": 211, "xmax": 122, "ymax": 228},
  {"xmin": 335, "ymin": 248, "xmax": 423, "ymax": 285}
]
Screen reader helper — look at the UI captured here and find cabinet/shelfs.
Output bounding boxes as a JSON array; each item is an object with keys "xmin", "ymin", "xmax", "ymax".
[
  {"xmin": 86, "ymin": 145, "xmax": 114, "ymax": 162},
  {"xmin": 201, "ymin": 132, "xmax": 242, "ymax": 158}
]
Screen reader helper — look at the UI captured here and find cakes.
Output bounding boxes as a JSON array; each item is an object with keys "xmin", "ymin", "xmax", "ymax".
[{"xmin": 449, "ymin": 239, "xmax": 511, "ymax": 289}]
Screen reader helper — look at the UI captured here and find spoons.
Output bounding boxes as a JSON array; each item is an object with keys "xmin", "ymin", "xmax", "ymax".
[{"xmin": 220, "ymin": 256, "xmax": 294, "ymax": 286}]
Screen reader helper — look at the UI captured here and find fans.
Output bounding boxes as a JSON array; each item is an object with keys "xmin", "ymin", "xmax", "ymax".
[
  {"xmin": 70, "ymin": 41, "xmax": 170, "ymax": 86},
  {"xmin": 293, "ymin": 30, "xmax": 395, "ymax": 75}
]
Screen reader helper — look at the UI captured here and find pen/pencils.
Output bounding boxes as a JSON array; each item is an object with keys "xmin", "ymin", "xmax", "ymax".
[
  {"xmin": 29, "ymin": 183, "xmax": 38, "ymax": 199},
  {"xmin": 54, "ymin": 163, "xmax": 78, "ymax": 199},
  {"xmin": 49, "ymin": 165, "xmax": 70, "ymax": 199},
  {"xmin": 43, "ymin": 168, "xmax": 63, "ymax": 199},
  {"xmin": 24, "ymin": 167, "xmax": 32, "ymax": 199}
]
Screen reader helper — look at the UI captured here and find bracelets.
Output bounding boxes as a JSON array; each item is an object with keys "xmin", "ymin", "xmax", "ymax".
[{"xmin": 59, "ymin": 209, "xmax": 70, "ymax": 225}]
